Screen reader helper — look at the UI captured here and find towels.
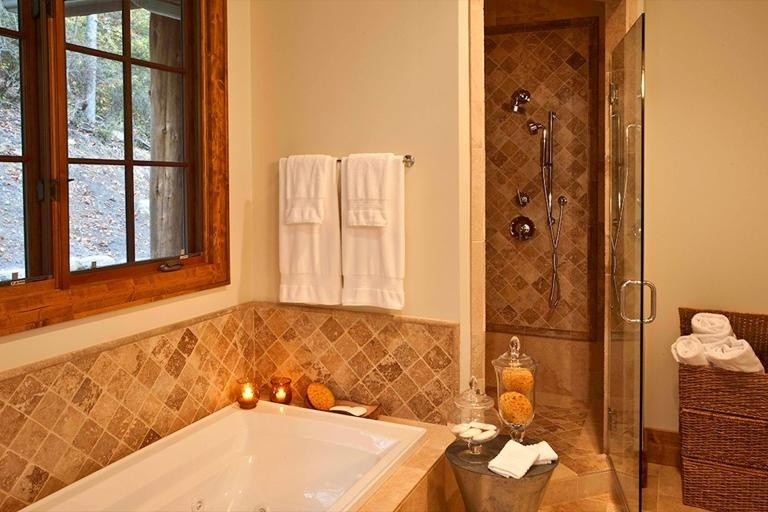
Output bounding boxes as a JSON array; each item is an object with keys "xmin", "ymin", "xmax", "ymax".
[
  {"xmin": 488, "ymin": 440, "xmax": 539, "ymax": 479},
  {"xmin": 691, "ymin": 312, "xmax": 735, "ymax": 336},
  {"xmin": 527, "ymin": 440, "xmax": 559, "ymax": 465},
  {"xmin": 707, "ymin": 336, "xmax": 765, "ymax": 373},
  {"xmin": 671, "ymin": 335, "xmax": 707, "ymax": 367},
  {"xmin": 346, "ymin": 155, "xmax": 385, "ymax": 226},
  {"xmin": 340, "ymin": 154, "xmax": 404, "ymax": 312},
  {"xmin": 278, "ymin": 153, "xmax": 340, "ymax": 305},
  {"xmin": 283, "ymin": 156, "xmax": 329, "ymax": 224}
]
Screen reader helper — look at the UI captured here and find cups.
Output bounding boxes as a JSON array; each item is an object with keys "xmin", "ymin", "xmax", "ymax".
[
  {"xmin": 268, "ymin": 376, "xmax": 294, "ymax": 405},
  {"xmin": 236, "ymin": 379, "xmax": 260, "ymax": 409}
]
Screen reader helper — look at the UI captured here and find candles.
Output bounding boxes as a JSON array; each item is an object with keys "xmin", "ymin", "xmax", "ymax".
[
  {"xmin": 276, "ymin": 389, "xmax": 288, "ymax": 403},
  {"xmin": 241, "ymin": 387, "xmax": 255, "ymax": 402}
]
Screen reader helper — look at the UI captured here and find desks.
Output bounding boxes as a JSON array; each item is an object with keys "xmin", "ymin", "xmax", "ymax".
[{"xmin": 445, "ymin": 433, "xmax": 559, "ymax": 511}]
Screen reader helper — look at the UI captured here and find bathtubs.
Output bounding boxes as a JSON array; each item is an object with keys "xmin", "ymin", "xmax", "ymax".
[{"xmin": 13, "ymin": 395, "xmax": 430, "ymax": 512}]
